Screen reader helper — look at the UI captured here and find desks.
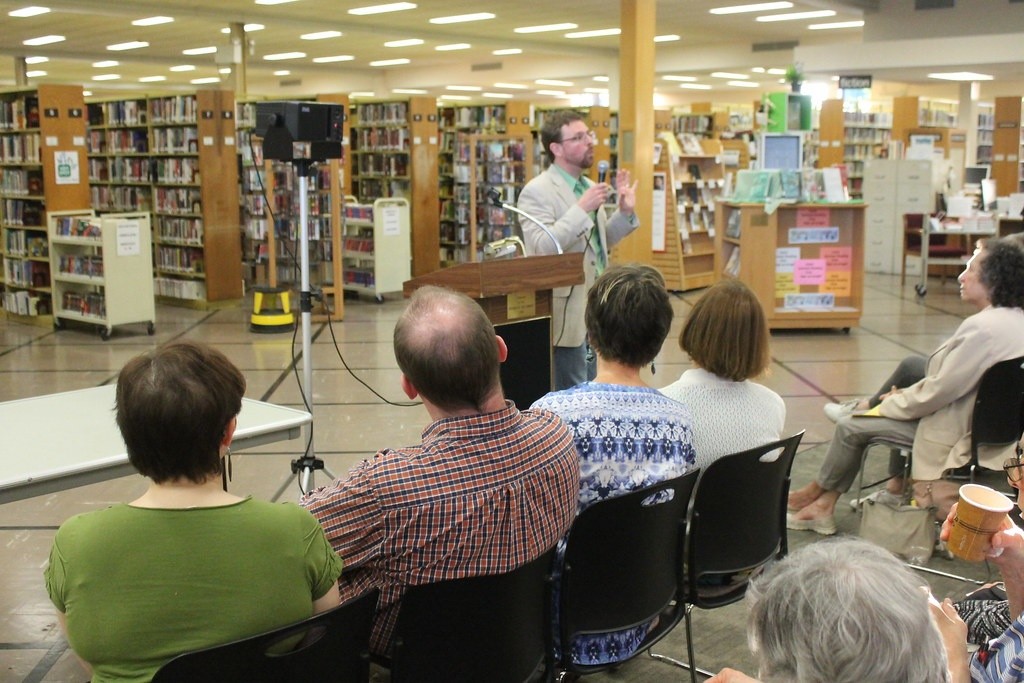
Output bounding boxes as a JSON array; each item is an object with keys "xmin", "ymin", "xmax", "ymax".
[{"xmin": 0, "ymin": 381, "xmax": 313, "ymax": 509}]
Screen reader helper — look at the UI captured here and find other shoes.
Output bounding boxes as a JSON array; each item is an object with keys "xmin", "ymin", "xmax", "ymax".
[{"xmin": 786, "ymin": 513, "xmax": 837, "ymax": 536}]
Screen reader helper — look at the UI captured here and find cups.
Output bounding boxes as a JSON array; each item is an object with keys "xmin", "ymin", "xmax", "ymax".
[{"xmin": 946, "ymin": 484, "xmax": 1014, "ymax": 563}]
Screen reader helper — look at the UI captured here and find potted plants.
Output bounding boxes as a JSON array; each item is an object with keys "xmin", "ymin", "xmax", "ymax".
[{"xmin": 785, "ymin": 62, "xmax": 804, "ymax": 92}]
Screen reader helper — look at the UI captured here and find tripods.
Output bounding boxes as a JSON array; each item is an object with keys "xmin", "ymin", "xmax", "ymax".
[{"xmin": 269, "ymin": 158, "xmax": 335, "ymax": 503}]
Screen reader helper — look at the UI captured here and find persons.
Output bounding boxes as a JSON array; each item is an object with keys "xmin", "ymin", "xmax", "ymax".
[
  {"xmin": 697, "ymin": 537, "xmax": 950, "ymax": 682},
  {"xmin": 528, "ymin": 263, "xmax": 696, "ymax": 665},
  {"xmin": 43, "ymin": 337, "xmax": 343, "ymax": 683},
  {"xmin": 654, "ymin": 278, "xmax": 787, "ymax": 591},
  {"xmin": 926, "ymin": 428, "xmax": 1024, "ymax": 682},
  {"xmin": 788, "ymin": 231, "xmax": 1024, "ymax": 534},
  {"xmin": 516, "ymin": 110, "xmax": 640, "ymax": 394},
  {"xmin": 294, "ymin": 283, "xmax": 583, "ymax": 683}
]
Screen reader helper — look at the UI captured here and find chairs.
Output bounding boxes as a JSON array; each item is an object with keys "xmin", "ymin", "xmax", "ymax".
[
  {"xmin": 850, "ymin": 352, "xmax": 1024, "ymax": 586},
  {"xmin": 150, "ymin": 426, "xmax": 808, "ymax": 683},
  {"xmin": 901, "ymin": 211, "xmax": 972, "ymax": 286}
]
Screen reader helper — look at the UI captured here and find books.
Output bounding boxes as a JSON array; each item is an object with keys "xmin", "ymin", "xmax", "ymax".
[{"xmin": 1, "ymin": 96, "xmax": 999, "ymax": 318}]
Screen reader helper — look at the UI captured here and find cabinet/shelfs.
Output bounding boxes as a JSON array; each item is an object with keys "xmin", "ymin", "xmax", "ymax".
[
  {"xmin": 893, "ymin": 95, "xmax": 994, "ymax": 167},
  {"xmin": 652, "ymin": 135, "xmax": 749, "ymax": 294},
  {"xmin": 0, "ymin": 84, "xmax": 90, "ymax": 329},
  {"xmin": 231, "ymin": 94, "xmax": 352, "ymax": 304},
  {"xmin": 714, "ymin": 198, "xmax": 869, "ymax": 335},
  {"xmin": 532, "ymin": 105, "xmax": 611, "ymax": 203},
  {"xmin": 46, "ymin": 209, "xmax": 156, "ymax": 338},
  {"xmin": 348, "ymin": 95, "xmax": 440, "ymax": 277},
  {"xmin": 343, "ymin": 194, "xmax": 413, "ymax": 302},
  {"xmin": 438, "ymin": 97, "xmax": 534, "ymax": 254},
  {"xmin": 819, "ymin": 99, "xmax": 892, "ymax": 199},
  {"xmin": 914, "ymin": 210, "xmax": 1000, "ymax": 297},
  {"xmin": 992, "ymin": 97, "xmax": 1024, "ymax": 195},
  {"xmin": 84, "ymin": 90, "xmax": 243, "ymax": 310}
]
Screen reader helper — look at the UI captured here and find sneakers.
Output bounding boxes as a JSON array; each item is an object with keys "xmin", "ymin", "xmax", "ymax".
[
  {"xmin": 850, "ymin": 489, "xmax": 905, "ymax": 511},
  {"xmin": 823, "ymin": 399, "xmax": 859, "ymax": 424}
]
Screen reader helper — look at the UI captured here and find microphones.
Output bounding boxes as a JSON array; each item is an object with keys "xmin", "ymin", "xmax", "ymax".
[{"xmin": 593, "ymin": 159, "xmax": 609, "ymax": 214}]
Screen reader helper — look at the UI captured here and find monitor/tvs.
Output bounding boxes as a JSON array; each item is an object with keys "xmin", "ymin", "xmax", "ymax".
[
  {"xmin": 964, "ymin": 165, "xmax": 991, "ymax": 187},
  {"xmin": 761, "ymin": 133, "xmax": 803, "ymax": 173}
]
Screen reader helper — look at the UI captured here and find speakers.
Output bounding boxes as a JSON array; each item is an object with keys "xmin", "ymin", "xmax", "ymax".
[{"xmin": 255, "ymin": 102, "xmax": 328, "ymax": 140}]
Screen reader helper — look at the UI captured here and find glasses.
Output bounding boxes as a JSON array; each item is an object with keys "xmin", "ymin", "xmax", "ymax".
[
  {"xmin": 556, "ymin": 129, "xmax": 593, "ymax": 143},
  {"xmin": 1003, "ymin": 458, "xmax": 1024, "ymax": 483}
]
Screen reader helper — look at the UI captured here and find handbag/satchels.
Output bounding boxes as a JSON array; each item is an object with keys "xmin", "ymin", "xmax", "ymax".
[{"xmin": 859, "ymin": 498, "xmax": 939, "ymax": 567}]
